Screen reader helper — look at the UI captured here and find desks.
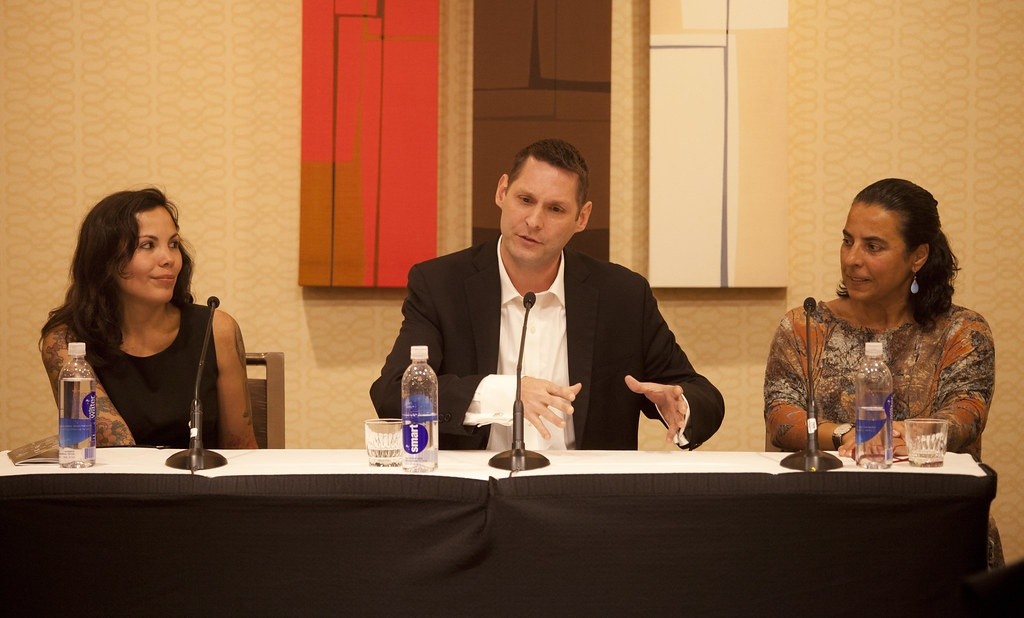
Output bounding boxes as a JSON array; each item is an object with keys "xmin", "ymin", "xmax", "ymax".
[{"xmin": 1, "ymin": 444, "xmax": 998, "ymax": 618}]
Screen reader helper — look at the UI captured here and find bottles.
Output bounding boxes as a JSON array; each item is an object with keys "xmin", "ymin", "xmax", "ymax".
[
  {"xmin": 59, "ymin": 341, "xmax": 96, "ymax": 468},
  {"xmin": 854, "ymin": 342, "xmax": 893, "ymax": 469},
  {"xmin": 402, "ymin": 345, "xmax": 439, "ymax": 472}
]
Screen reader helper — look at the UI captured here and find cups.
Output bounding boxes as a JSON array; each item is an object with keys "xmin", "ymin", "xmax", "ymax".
[
  {"xmin": 364, "ymin": 419, "xmax": 402, "ymax": 467},
  {"xmin": 905, "ymin": 418, "xmax": 948, "ymax": 469}
]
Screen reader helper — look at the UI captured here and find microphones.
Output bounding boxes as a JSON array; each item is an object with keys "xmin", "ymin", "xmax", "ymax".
[
  {"xmin": 165, "ymin": 295, "xmax": 229, "ymax": 470},
  {"xmin": 488, "ymin": 291, "xmax": 551, "ymax": 471},
  {"xmin": 779, "ymin": 296, "xmax": 843, "ymax": 471}
]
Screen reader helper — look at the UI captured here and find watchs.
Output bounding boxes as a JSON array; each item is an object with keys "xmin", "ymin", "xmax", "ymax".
[{"xmin": 833, "ymin": 423, "xmax": 855, "ymax": 451}]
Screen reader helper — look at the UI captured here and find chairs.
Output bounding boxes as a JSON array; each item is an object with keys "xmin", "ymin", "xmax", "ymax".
[{"xmin": 241, "ymin": 350, "xmax": 286, "ymax": 452}]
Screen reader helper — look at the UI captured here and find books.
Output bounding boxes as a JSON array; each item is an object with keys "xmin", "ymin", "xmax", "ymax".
[{"xmin": 8, "ymin": 435, "xmax": 59, "ymax": 466}]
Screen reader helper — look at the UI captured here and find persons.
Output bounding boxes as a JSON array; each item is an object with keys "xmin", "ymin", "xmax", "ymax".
[
  {"xmin": 370, "ymin": 138, "xmax": 726, "ymax": 450},
  {"xmin": 40, "ymin": 188, "xmax": 258, "ymax": 449},
  {"xmin": 764, "ymin": 178, "xmax": 1005, "ymax": 573}
]
